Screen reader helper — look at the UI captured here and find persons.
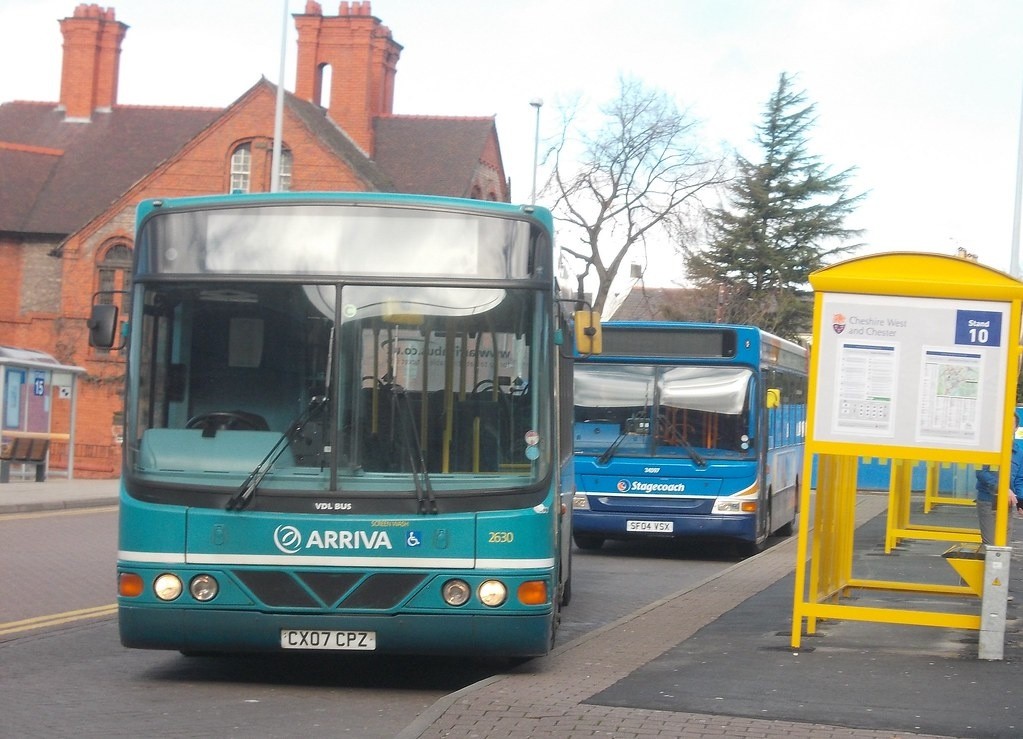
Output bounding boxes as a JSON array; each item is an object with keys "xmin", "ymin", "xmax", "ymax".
[{"xmin": 958, "ymin": 412, "xmax": 1023, "ymax": 600}]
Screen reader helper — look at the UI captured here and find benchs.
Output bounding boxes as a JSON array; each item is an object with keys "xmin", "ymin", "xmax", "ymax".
[{"xmin": 0, "ymin": 437, "xmax": 52, "ymax": 483}]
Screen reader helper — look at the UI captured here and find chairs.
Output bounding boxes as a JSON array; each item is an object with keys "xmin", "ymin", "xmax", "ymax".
[{"xmin": 143, "ymin": 376, "xmax": 806, "ymax": 473}]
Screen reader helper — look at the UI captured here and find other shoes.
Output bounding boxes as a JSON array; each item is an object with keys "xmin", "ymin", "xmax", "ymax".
[
  {"xmin": 959, "ymin": 577, "xmax": 969, "ymax": 586},
  {"xmin": 1007, "ymin": 595, "xmax": 1015, "ymax": 601}
]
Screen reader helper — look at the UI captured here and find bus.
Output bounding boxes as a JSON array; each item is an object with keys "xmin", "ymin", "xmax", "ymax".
[
  {"xmin": 568, "ymin": 318, "xmax": 812, "ymax": 556},
  {"xmin": 84, "ymin": 187, "xmax": 574, "ymax": 674}
]
